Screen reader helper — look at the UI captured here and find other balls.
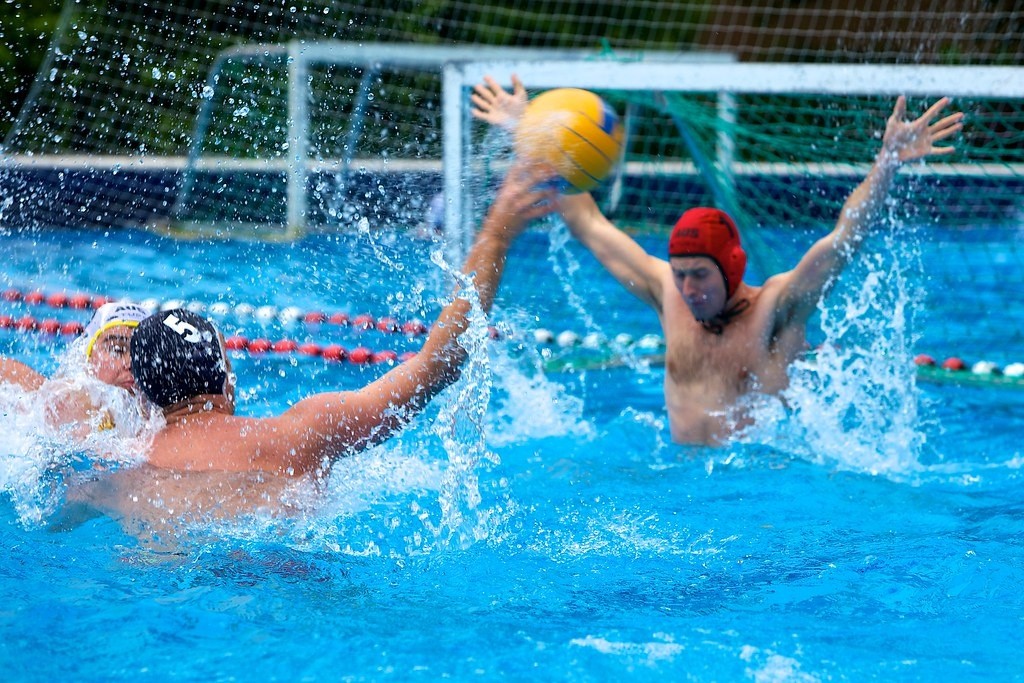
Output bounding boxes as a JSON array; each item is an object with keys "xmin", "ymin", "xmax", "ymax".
[{"xmin": 512, "ymin": 88, "xmax": 624, "ymax": 194}]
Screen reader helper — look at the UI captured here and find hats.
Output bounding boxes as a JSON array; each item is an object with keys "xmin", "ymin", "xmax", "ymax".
[
  {"xmin": 668, "ymin": 207, "xmax": 747, "ymax": 301},
  {"xmin": 83, "ymin": 301, "xmax": 149, "ymax": 357},
  {"xmin": 129, "ymin": 308, "xmax": 227, "ymax": 407}
]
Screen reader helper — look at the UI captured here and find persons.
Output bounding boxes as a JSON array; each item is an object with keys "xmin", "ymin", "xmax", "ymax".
[
  {"xmin": 0, "ymin": 302, "xmax": 168, "ymax": 445},
  {"xmin": 127, "ymin": 155, "xmax": 558, "ymax": 474},
  {"xmin": 471, "ymin": 71, "xmax": 965, "ymax": 449}
]
